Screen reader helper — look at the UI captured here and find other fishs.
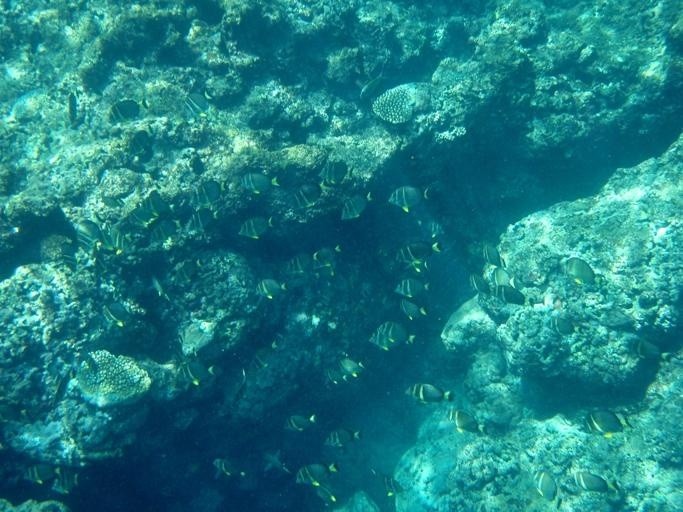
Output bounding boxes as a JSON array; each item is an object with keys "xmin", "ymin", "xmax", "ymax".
[{"xmin": 0, "ymin": 54, "xmax": 682, "ymax": 512}]
